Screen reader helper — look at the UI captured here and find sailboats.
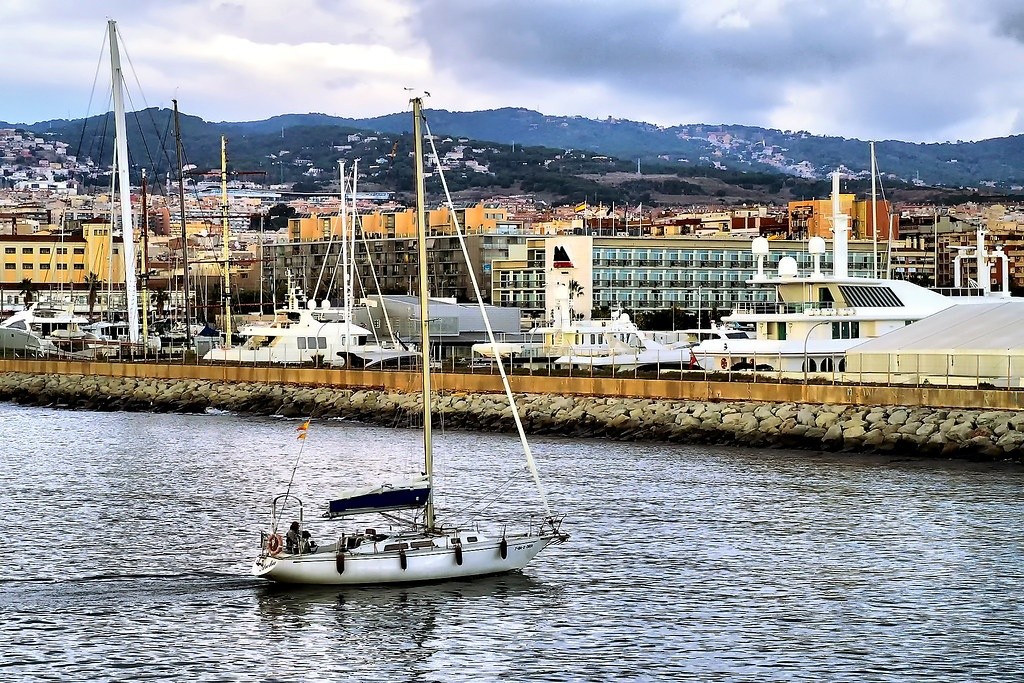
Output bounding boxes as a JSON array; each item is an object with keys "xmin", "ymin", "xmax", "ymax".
[
  {"xmin": 0, "ymin": 18, "xmax": 1023, "ymax": 396},
  {"xmin": 253, "ymin": 85, "xmax": 566, "ymax": 583}
]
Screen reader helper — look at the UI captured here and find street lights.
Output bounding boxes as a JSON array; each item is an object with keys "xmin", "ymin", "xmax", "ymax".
[{"xmin": 804, "ymin": 321, "xmax": 831, "ymax": 383}]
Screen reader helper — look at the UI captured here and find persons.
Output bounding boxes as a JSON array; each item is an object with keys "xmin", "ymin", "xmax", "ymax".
[{"xmin": 286, "ymin": 521, "xmax": 301, "ymax": 550}]
